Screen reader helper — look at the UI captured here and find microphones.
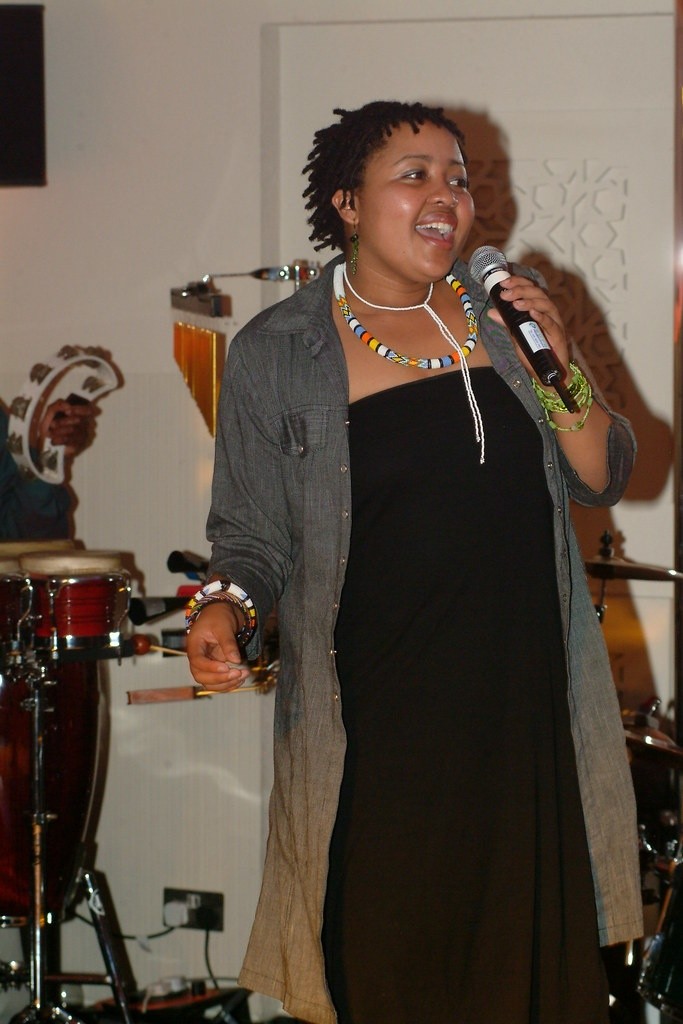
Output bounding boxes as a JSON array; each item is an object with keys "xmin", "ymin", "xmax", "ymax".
[{"xmin": 467, "ymin": 246, "xmax": 576, "ymax": 415}]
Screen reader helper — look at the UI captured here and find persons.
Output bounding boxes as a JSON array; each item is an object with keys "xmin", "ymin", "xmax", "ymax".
[{"xmin": 182, "ymin": 102, "xmax": 644, "ymax": 1024}]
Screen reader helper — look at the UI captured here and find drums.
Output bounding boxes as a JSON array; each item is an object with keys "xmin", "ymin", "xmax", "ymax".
[
  {"xmin": 19, "ymin": 548, "xmax": 131, "ymax": 651},
  {"xmin": 1, "ymin": 538, "xmax": 101, "ymax": 927}
]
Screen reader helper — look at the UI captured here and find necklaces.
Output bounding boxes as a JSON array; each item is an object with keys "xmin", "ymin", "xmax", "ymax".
[
  {"xmin": 334, "ymin": 261, "xmax": 479, "ymax": 371},
  {"xmin": 343, "ymin": 261, "xmax": 485, "ymax": 465}
]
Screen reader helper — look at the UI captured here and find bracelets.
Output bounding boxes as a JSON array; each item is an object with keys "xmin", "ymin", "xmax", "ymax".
[
  {"xmin": 531, "ymin": 361, "xmax": 595, "ymax": 432},
  {"xmin": 186, "ymin": 593, "xmax": 250, "ymax": 647},
  {"xmin": 185, "ymin": 579, "xmax": 255, "ymax": 636}
]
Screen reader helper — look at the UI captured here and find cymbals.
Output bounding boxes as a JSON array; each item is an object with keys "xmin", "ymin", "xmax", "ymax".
[
  {"xmin": 579, "ymin": 555, "xmax": 682, "ymax": 582},
  {"xmin": 4, "ymin": 345, "xmax": 118, "ymax": 485},
  {"xmin": 615, "ymin": 725, "xmax": 682, "ymax": 759}
]
